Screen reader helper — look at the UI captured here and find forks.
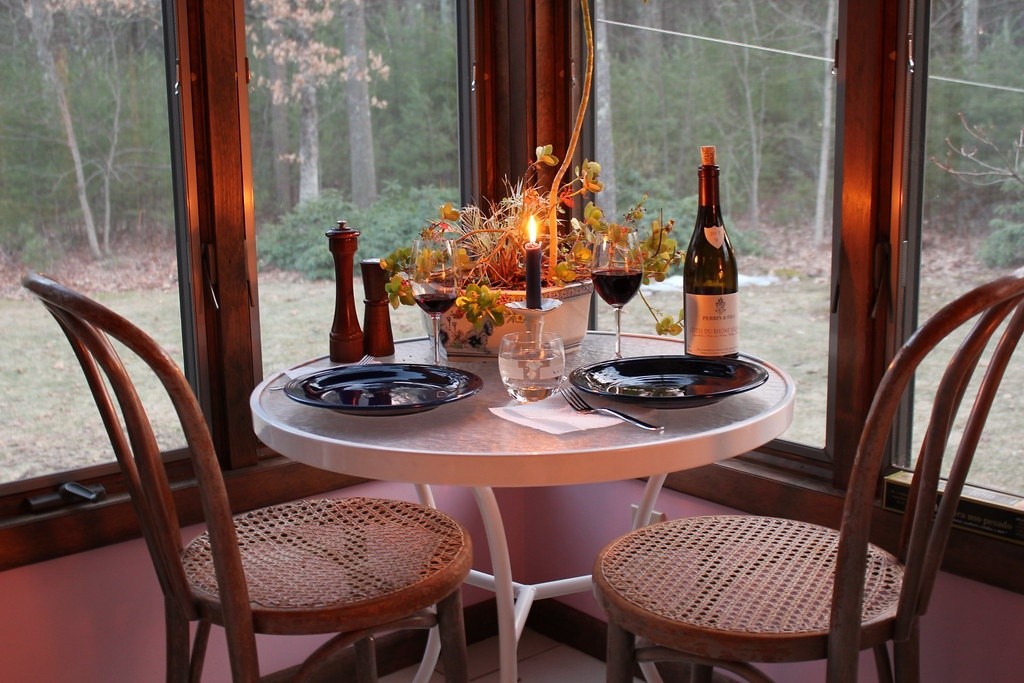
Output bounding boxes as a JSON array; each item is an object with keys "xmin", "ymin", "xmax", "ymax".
[
  {"xmin": 558, "ymin": 386, "xmax": 660, "ymax": 436},
  {"xmin": 268, "ymin": 356, "xmax": 375, "ymax": 393}
]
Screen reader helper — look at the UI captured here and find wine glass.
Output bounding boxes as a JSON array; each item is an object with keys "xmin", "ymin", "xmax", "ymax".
[
  {"xmin": 410, "ymin": 239, "xmax": 457, "ymax": 365},
  {"xmin": 588, "ymin": 231, "xmax": 645, "ymax": 358}
]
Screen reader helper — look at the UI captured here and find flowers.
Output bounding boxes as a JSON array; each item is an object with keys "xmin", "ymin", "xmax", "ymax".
[{"xmin": 380, "ymin": 1, "xmax": 691, "ymax": 335}]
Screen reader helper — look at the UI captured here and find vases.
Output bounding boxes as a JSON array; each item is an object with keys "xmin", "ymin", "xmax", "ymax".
[{"xmin": 424, "ymin": 266, "xmax": 597, "ymax": 361}]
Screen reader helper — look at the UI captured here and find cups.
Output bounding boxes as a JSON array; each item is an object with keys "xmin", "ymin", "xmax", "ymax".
[{"xmin": 498, "ymin": 331, "xmax": 565, "ymax": 405}]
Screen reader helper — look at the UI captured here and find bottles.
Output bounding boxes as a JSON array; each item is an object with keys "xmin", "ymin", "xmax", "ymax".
[
  {"xmin": 282, "ymin": 360, "xmax": 484, "ymax": 418},
  {"xmin": 679, "ymin": 165, "xmax": 742, "ymax": 361}
]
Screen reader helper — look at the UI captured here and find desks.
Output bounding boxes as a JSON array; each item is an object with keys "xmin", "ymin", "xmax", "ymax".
[{"xmin": 249, "ymin": 329, "xmax": 797, "ymax": 683}]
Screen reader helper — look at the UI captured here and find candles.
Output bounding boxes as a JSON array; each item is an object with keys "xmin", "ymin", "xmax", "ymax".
[{"xmin": 522, "ymin": 212, "xmax": 544, "ymax": 362}]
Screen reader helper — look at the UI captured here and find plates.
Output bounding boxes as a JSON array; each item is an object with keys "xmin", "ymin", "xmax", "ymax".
[{"xmin": 570, "ymin": 354, "xmax": 769, "ymax": 410}]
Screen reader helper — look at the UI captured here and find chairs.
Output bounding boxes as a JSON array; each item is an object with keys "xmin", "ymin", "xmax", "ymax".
[
  {"xmin": 20, "ymin": 273, "xmax": 473, "ymax": 683},
  {"xmin": 591, "ymin": 263, "xmax": 1024, "ymax": 682}
]
